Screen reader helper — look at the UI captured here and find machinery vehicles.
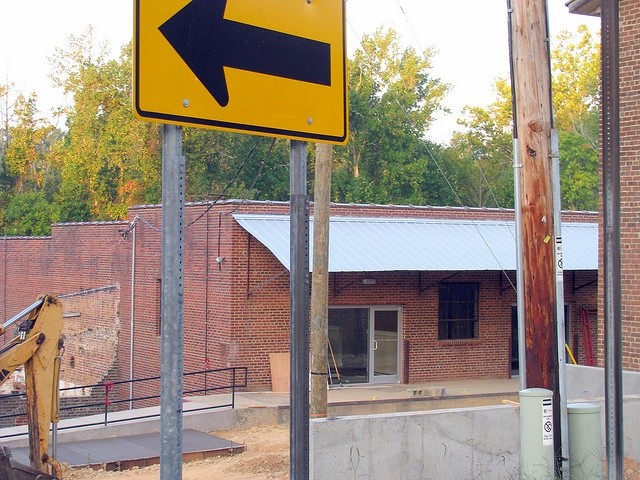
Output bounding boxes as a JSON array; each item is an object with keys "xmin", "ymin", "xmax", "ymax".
[{"xmin": 1, "ymin": 291, "xmax": 66, "ymax": 480}]
